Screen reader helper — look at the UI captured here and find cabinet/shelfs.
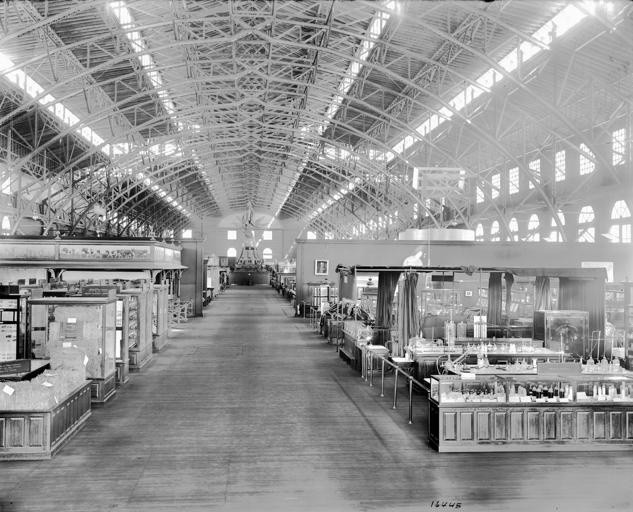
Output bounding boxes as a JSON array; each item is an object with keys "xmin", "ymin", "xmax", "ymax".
[
  {"xmin": 0, "ymin": 278, "xmax": 171, "ymax": 461},
  {"xmin": 338, "ymin": 320, "xmax": 367, "ymax": 369},
  {"xmin": 428, "ymin": 373, "xmax": 633, "ymax": 453},
  {"xmin": 403, "ymin": 345, "xmax": 571, "ymax": 396},
  {"xmin": 534, "ymin": 310, "xmax": 589, "ymax": 362}
]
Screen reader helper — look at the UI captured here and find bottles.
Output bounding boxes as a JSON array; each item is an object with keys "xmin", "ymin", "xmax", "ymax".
[
  {"xmin": 464, "ymin": 341, "xmax": 625, "ymax": 374},
  {"xmin": 508, "ymin": 383, "xmax": 628, "ymax": 402}
]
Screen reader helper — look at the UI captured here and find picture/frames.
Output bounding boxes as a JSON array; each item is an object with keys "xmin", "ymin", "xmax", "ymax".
[{"xmin": 315, "ymin": 259, "xmax": 329, "ymax": 275}]
